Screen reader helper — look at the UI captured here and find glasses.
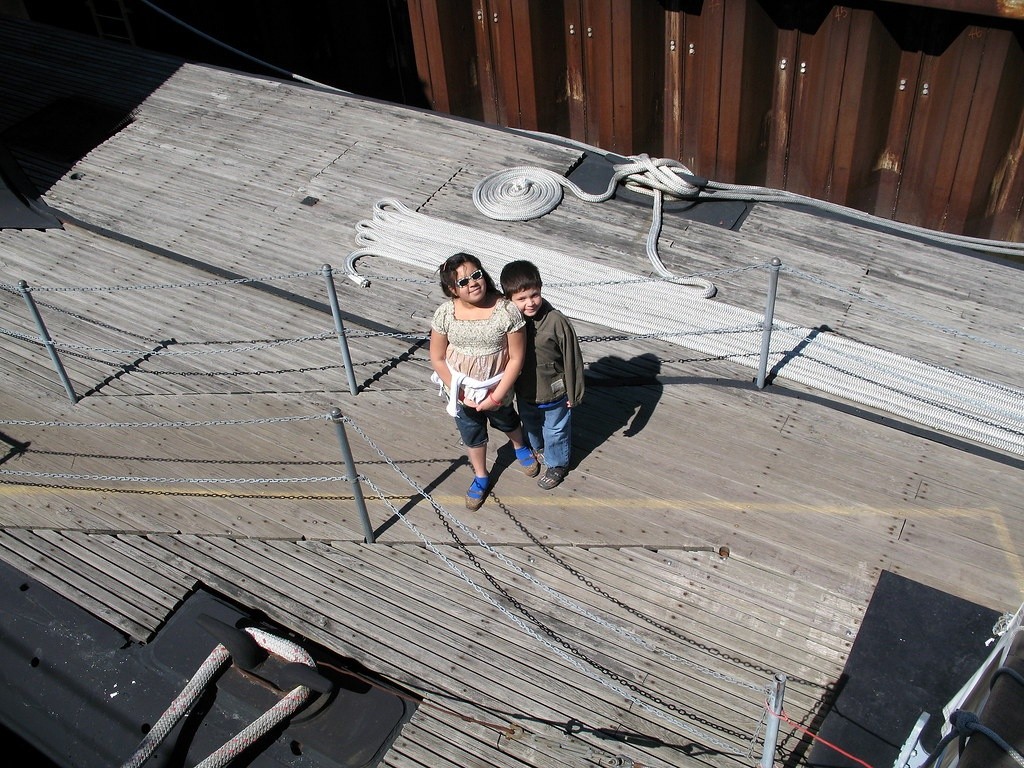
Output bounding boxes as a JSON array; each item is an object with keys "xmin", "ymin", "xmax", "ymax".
[{"xmin": 455, "ymin": 270, "xmax": 483, "ymax": 288}]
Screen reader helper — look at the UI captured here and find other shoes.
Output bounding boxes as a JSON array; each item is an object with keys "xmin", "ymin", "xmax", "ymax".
[
  {"xmin": 534, "ymin": 450, "xmax": 546, "ymax": 464},
  {"xmin": 538, "ymin": 468, "xmax": 568, "ymax": 490},
  {"xmin": 465, "ymin": 471, "xmax": 493, "ymax": 511},
  {"xmin": 514, "ymin": 447, "xmax": 539, "ymax": 476}
]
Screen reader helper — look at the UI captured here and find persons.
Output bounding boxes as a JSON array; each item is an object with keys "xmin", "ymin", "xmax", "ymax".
[
  {"xmin": 499, "ymin": 259, "xmax": 586, "ymax": 490},
  {"xmin": 430, "ymin": 252, "xmax": 539, "ymax": 511}
]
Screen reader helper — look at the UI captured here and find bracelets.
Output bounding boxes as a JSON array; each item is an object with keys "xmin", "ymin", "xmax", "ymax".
[{"xmin": 490, "ymin": 392, "xmax": 502, "ymax": 406}]
